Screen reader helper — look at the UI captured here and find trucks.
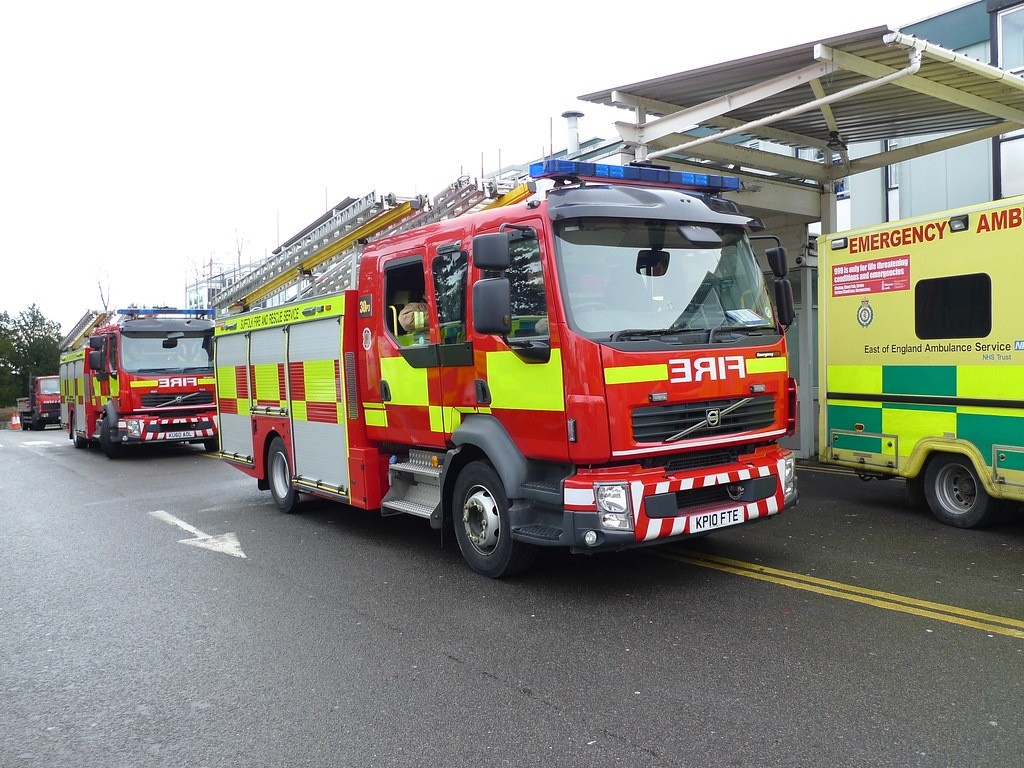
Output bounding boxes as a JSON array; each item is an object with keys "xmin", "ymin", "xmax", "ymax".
[
  {"xmin": 812, "ymin": 189, "xmax": 1024, "ymax": 530},
  {"xmin": 16, "ymin": 373, "xmax": 62, "ymax": 431}
]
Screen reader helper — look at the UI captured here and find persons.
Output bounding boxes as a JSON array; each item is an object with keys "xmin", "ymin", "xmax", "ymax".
[
  {"xmin": 115, "ymin": 344, "xmax": 149, "ymax": 369},
  {"xmin": 399, "ymin": 281, "xmax": 448, "ymax": 333},
  {"xmin": 503, "ymin": 257, "xmax": 549, "ymax": 336}
]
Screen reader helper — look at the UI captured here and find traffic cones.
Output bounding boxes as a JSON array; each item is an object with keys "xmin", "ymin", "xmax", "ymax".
[{"xmin": 10, "ymin": 410, "xmax": 23, "ymax": 431}]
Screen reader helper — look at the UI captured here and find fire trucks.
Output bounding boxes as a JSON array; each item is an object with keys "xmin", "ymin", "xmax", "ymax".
[
  {"xmin": 56, "ymin": 305, "xmax": 223, "ymax": 459},
  {"xmin": 210, "ymin": 153, "xmax": 804, "ymax": 584}
]
